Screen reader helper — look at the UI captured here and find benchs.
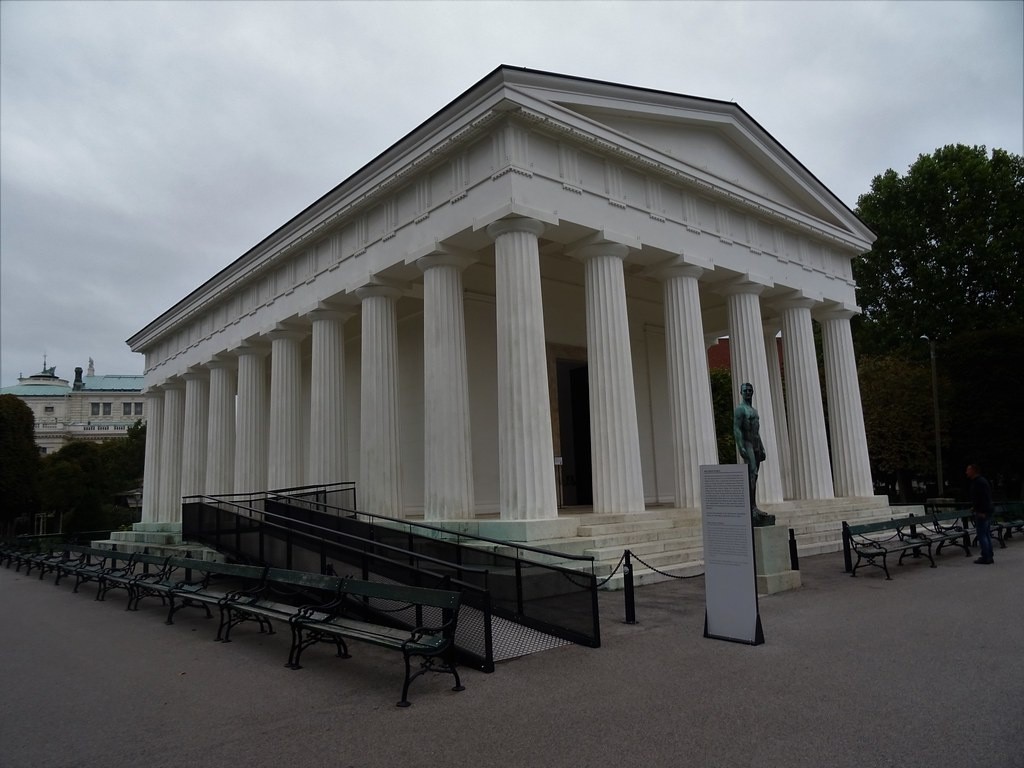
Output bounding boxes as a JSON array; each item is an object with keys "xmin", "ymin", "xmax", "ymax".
[
  {"xmin": 842, "ymin": 503, "xmax": 1024, "ymax": 581},
  {"xmin": 1, "ymin": 537, "xmax": 465, "ymax": 708}
]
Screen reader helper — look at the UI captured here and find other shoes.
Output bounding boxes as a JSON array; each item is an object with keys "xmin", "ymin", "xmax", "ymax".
[{"xmin": 973, "ymin": 557, "xmax": 994, "ymax": 564}]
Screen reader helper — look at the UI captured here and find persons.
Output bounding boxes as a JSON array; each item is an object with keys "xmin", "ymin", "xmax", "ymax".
[
  {"xmin": 734, "ymin": 383, "xmax": 768, "ymax": 518},
  {"xmin": 966, "ymin": 465, "xmax": 994, "ymax": 564}
]
